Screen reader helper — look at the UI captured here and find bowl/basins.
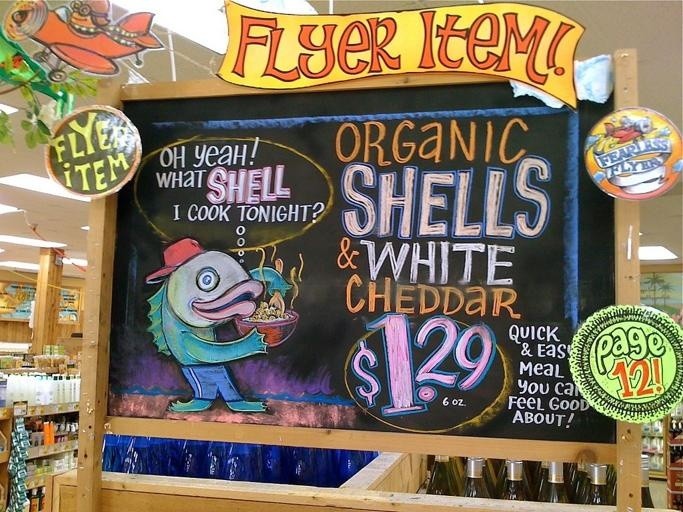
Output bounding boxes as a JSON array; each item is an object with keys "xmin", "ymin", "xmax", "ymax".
[{"xmin": 236, "ymin": 311, "xmax": 300, "ymax": 351}]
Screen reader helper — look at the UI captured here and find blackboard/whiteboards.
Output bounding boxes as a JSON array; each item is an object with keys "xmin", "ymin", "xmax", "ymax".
[{"xmin": 80, "ymin": 47, "xmax": 641, "ymax": 465}]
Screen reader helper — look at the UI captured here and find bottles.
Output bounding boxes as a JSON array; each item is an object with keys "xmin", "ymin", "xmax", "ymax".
[
  {"xmin": 101, "ymin": 430, "xmax": 379, "ymax": 486},
  {"xmin": 1, "ymin": 373, "xmax": 81, "ymax": 408},
  {"xmin": 419, "ymin": 455, "xmax": 614, "ymax": 503}
]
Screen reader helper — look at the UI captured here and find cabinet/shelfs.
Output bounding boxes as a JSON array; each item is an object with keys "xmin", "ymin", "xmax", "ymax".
[
  {"xmin": 0, "ymin": 401, "xmax": 79, "ymax": 512},
  {"xmin": 661, "ymin": 413, "xmax": 683, "ymax": 512}
]
